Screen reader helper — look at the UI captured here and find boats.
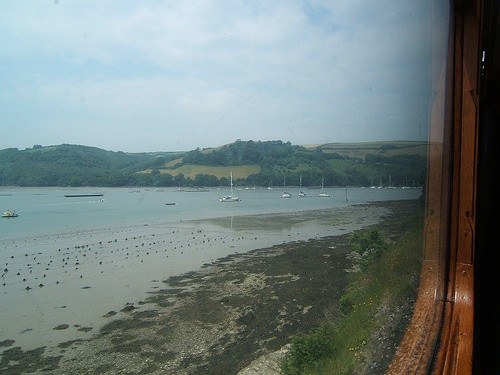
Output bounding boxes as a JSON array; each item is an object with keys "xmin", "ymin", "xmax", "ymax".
[
  {"xmin": 267, "ymin": 181, "xmax": 276, "ymax": 189},
  {"xmin": 165, "ymin": 202, "xmax": 175, "ymax": 205},
  {"xmin": 2, "ymin": 208, "xmax": 21, "ymax": 217}
]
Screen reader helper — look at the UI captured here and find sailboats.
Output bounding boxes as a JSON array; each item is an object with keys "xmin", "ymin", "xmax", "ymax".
[
  {"xmin": 281, "ymin": 175, "xmax": 292, "ymax": 198},
  {"xmin": 369, "ymin": 175, "xmax": 424, "ymax": 190},
  {"xmin": 320, "ymin": 176, "xmax": 330, "ymax": 197},
  {"xmin": 297, "ymin": 175, "xmax": 307, "ymax": 197},
  {"xmin": 220, "ymin": 171, "xmax": 241, "ymax": 202}
]
